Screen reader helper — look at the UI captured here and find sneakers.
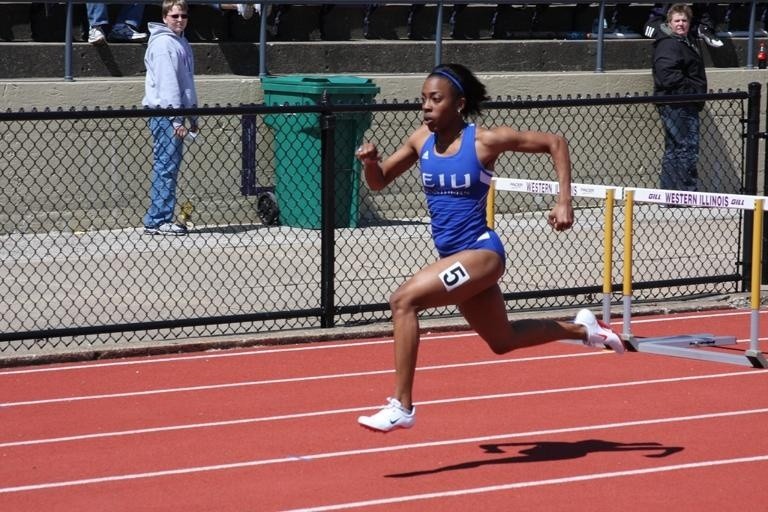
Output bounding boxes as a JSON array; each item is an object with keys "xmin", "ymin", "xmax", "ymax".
[
  {"xmin": 698, "ymin": 24, "xmax": 724, "ymax": 48},
  {"xmin": 236, "ymin": 3, "xmax": 253, "ymax": 19},
  {"xmin": 575, "ymin": 307, "xmax": 626, "ymax": 353},
  {"xmin": 88, "ymin": 27, "xmax": 108, "ymax": 45},
  {"xmin": 111, "ymin": 26, "xmax": 148, "ymax": 42},
  {"xmin": 143, "ymin": 221, "xmax": 186, "ymax": 235},
  {"xmin": 357, "ymin": 400, "xmax": 417, "ymax": 433},
  {"xmin": 255, "ymin": 4, "xmax": 261, "ymax": 16}
]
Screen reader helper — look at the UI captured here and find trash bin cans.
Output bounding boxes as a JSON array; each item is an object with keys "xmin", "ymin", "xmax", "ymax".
[{"xmin": 257, "ymin": 74, "xmax": 380, "ymax": 229}]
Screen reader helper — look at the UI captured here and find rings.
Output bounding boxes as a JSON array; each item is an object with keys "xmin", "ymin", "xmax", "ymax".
[{"xmin": 357, "ymin": 145, "xmax": 363, "ymax": 152}]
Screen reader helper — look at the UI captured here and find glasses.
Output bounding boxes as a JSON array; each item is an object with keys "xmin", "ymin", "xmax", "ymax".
[{"xmin": 166, "ymin": 14, "xmax": 187, "ymax": 18}]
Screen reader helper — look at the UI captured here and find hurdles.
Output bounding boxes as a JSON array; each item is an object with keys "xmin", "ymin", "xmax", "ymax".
[
  {"xmin": 485, "ymin": 176, "xmax": 714, "ymax": 351},
  {"xmin": 622, "ymin": 177, "xmax": 767, "ymax": 368}
]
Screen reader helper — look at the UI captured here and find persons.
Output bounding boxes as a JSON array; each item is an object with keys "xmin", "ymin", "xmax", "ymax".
[
  {"xmin": 184, "ymin": 0, "xmax": 281, "ymax": 42},
  {"xmin": 353, "ymin": 60, "xmax": 627, "ymax": 433},
  {"xmin": 138, "ymin": 1, "xmax": 201, "ymax": 237},
  {"xmin": 83, "ymin": 0, "xmax": 148, "ymax": 45},
  {"xmin": 650, "ymin": 2, "xmax": 708, "ymax": 208},
  {"xmin": 642, "ymin": 1, "xmax": 724, "ymax": 49}
]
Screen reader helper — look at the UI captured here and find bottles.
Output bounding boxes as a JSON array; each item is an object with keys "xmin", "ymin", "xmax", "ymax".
[
  {"xmin": 758, "ymin": 42, "xmax": 767, "ymax": 70},
  {"xmin": 176, "ymin": 206, "xmax": 188, "ymax": 228}
]
